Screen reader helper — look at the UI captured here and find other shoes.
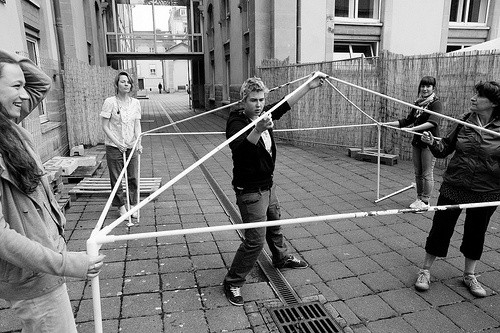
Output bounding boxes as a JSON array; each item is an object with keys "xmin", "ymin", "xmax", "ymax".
[
  {"xmin": 131, "ymin": 206, "xmax": 137, "ymax": 218},
  {"xmin": 118, "ymin": 205, "xmax": 128, "ymax": 220}
]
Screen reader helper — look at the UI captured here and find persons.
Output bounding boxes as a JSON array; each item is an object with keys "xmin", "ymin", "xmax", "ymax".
[
  {"xmin": 415, "ymin": 80, "xmax": 500, "ymax": 297},
  {"xmin": 158, "ymin": 81, "xmax": 162, "ymax": 93},
  {"xmin": 0, "ymin": 51, "xmax": 105, "ymax": 333},
  {"xmin": 99, "ymin": 71, "xmax": 142, "ymax": 221},
  {"xmin": 377, "ymin": 75, "xmax": 442, "ymax": 214},
  {"xmin": 223, "ymin": 69, "xmax": 329, "ymax": 306}
]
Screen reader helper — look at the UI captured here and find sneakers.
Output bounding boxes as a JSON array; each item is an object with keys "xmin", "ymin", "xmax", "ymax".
[
  {"xmin": 463, "ymin": 274, "xmax": 486, "ymax": 297},
  {"xmin": 225, "ymin": 286, "xmax": 243, "ymax": 305},
  {"xmin": 409, "ymin": 199, "xmax": 430, "ymax": 213},
  {"xmin": 280, "ymin": 256, "xmax": 308, "ymax": 268},
  {"xmin": 415, "ymin": 269, "xmax": 430, "ymax": 290}
]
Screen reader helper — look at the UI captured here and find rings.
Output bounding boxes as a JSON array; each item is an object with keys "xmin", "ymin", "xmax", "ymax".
[{"xmin": 94, "ymin": 264, "xmax": 98, "ymax": 269}]
